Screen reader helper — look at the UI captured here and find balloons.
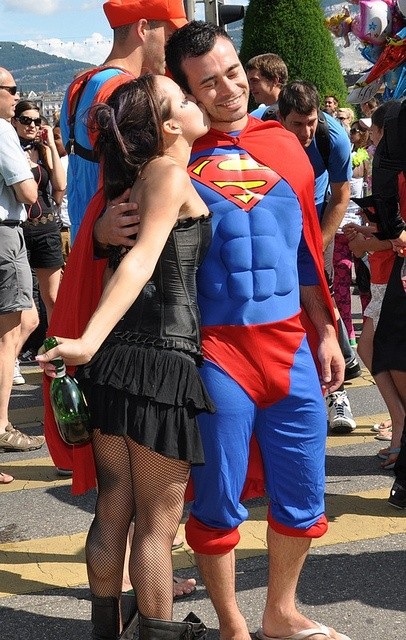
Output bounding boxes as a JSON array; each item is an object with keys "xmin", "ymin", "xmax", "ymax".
[{"xmin": 323, "ymin": 0, "xmax": 406, "ymax": 102}]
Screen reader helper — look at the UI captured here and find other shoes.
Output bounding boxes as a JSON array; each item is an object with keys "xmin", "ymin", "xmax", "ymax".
[
  {"xmin": 344, "ymin": 363, "xmax": 362, "ymax": 381},
  {"xmin": 349, "ymin": 339, "xmax": 358, "ymax": 348},
  {"xmin": 387, "ymin": 483, "xmax": 406, "ymax": 510}
]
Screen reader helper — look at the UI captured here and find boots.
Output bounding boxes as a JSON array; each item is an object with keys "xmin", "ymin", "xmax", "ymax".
[
  {"xmin": 137, "ymin": 609, "xmax": 209, "ymax": 640},
  {"xmin": 90, "ymin": 595, "xmax": 141, "ymax": 640}
]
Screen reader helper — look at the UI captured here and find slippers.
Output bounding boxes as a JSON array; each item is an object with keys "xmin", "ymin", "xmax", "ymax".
[{"xmin": 258, "ymin": 620, "xmax": 353, "ymax": 640}]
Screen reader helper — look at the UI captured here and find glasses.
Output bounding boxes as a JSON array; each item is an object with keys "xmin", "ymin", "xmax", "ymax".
[
  {"xmin": 336, "ymin": 116, "xmax": 349, "ymax": 120},
  {"xmin": 0, "ymin": 85, "xmax": 17, "ymax": 95},
  {"xmin": 350, "ymin": 127, "xmax": 359, "ymax": 134},
  {"xmin": 16, "ymin": 116, "xmax": 42, "ymax": 126}
]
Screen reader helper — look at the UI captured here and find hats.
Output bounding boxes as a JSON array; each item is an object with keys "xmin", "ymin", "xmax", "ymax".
[
  {"xmin": 102, "ymin": 0, "xmax": 189, "ymax": 29},
  {"xmin": 359, "ymin": 118, "xmax": 372, "ymax": 129}
]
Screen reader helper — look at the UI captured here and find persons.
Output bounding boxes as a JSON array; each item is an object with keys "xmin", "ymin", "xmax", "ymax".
[
  {"xmin": 59, "ymin": 1, "xmax": 199, "ymax": 600},
  {"xmin": 0, "ymin": 52, "xmax": 406, "ymax": 602},
  {"xmin": 36, "ymin": 75, "xmax": 211, "ymax": 640},
  {"xmin": 91, "ymin": 18, "xmax": 351, "ymax": 640}
]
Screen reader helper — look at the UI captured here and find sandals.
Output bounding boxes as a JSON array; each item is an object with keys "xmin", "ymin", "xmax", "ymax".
[
  {"xmin": 171, "ymin": 541, "xmax": 184, "ymax": 551},
  {"xmin": 172, "ymin": 577, "xmax": 198, "ymax": 601},
  {"xmin": 0, "ymin": 471, "xmax": 15, "ymax": 484},
  {"xmin": 373, "ymin": 418, "xmax": 392, "ymax": 441},
  {"xmin": 376, "ymin": 446, "xmax": 401, "ymax": 469}
]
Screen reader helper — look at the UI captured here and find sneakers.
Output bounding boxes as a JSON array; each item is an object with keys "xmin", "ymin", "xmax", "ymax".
[
  {"xmin": 13, "ymin": 361, "xmax": 26, "ymax": 385},
  {"xmin": 0, "ymin": 422, "xmax": 45, "ymax": 453},
  {"xmin": 326, "ymin": 389, "xmax": 357, "ymax": 434},
  {"xmin": 18, "ymin": 349, "xmax": 38, "ymax": 364}
]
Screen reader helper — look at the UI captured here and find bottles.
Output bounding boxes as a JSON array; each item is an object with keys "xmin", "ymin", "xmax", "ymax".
[{"xmin": 45, "ymin": 336, "xmax": 91, "ymax": 447}]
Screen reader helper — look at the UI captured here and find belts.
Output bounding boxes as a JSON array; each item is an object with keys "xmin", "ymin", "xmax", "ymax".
[
  {"xmin": 59, "ymin": 227, "xmax": 70, "ymax": 232},
  {"xmin": 0, "ymin": 219, "xmax": 21, "ymax": 226}
]
